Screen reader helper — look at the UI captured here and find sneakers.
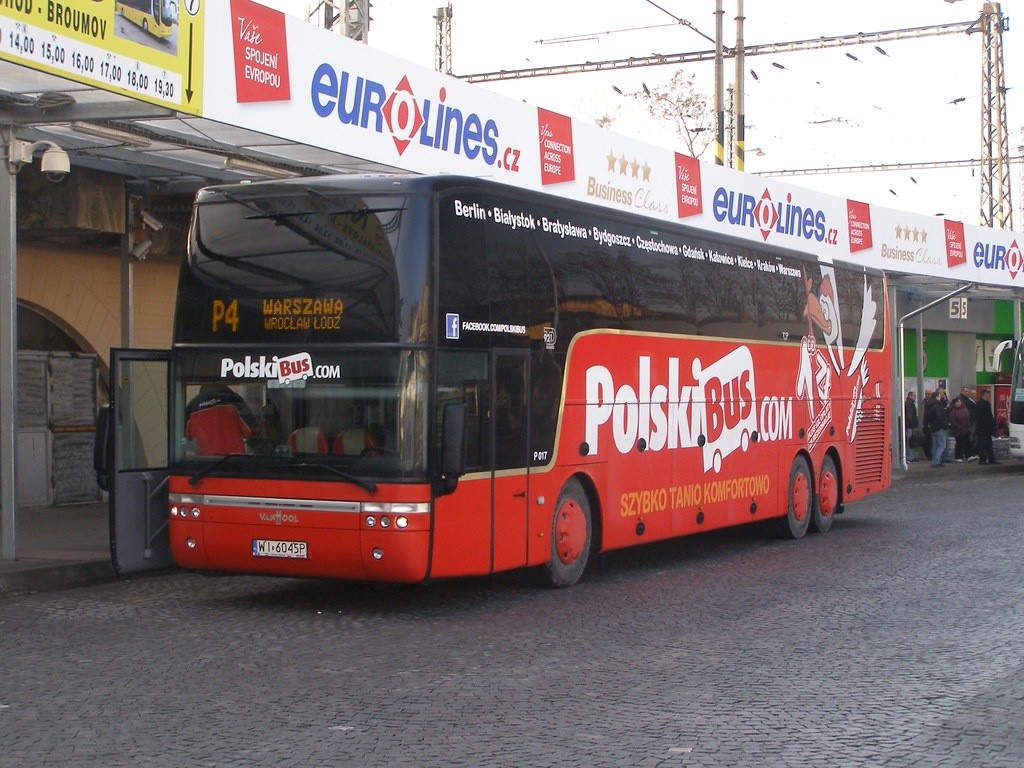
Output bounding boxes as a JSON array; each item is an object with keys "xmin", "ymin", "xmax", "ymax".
[
  {"xmin": 967, "ymin": 455, "xmax": 978, "ymax": 463},
  {"xmin": 956, "ymin": 457, "xmax": 965, "ymax": 463}
]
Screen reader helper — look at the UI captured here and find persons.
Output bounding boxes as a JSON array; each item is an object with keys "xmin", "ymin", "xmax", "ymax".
[
  {"xmin": 905, "ymin": 391, "xmax": 920, "ymax": 464},
  {"xmin": 935, "ymin": 386, "xmax": 952, "ymax": 463},
  {"xmin": 920, "ymin": 388, "xmax": 933, "ymax": 460},
  {"xmin": 926, "ymin": 391, "xmax": 951, "ymax": 468},
  {"xmin": 184, "ymin": 383, "xmax": 256, "ymax": 456},
  {"xmin": 949, "ymin": 398, "xmax": 977, "ymax": 463},
  {"xmin": 973, "ymin": 389, "xmax": 1003, "ymax": 466},
  {"xmin": 955, "ymin": 386, "xmax": 971, "ymax": 404},
  {"xmin": 966, "ymin": 388, "xmax": 982, "ymax": 459}
]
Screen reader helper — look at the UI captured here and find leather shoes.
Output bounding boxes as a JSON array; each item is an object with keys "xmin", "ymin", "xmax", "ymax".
[
  {"xmin": 980, "ymin": 458, "xmax": 993, "ymax": 465},
  {"xmin": 990, "ymin": 459, "xmax": 1001, "ymax": 466}
]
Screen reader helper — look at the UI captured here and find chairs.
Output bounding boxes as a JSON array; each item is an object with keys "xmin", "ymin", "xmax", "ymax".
[
  {"xmin": 288, "ymin": 427, "xmax": 330, "ymax": 454},
  {"xmin": 332, "ymin": 426, "xmax": 379, "ymax": 457}
]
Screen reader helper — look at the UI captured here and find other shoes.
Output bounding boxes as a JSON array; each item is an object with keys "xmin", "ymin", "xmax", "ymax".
[{"xmin": 907, "ymin": 458, "xmax": 917, "ymax": 464}]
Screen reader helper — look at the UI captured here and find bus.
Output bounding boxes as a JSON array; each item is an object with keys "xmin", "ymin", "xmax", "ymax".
[{"xmin": 171, "ymin": 173, "xmax": 893, "ymax": 592}]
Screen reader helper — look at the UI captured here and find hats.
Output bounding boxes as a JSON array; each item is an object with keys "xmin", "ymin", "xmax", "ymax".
[{"xmin": 979, "ymin": 388, "xmax": 991, "ymax": 395}]
